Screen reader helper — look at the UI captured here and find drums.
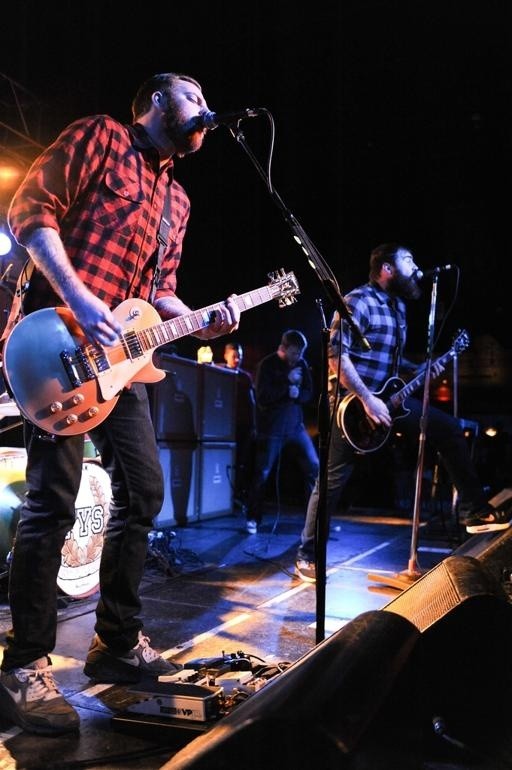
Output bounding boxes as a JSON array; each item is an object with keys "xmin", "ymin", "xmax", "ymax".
[{"xmin": 0, "ymin": 446, "xmax": 113, "ymax": 600}]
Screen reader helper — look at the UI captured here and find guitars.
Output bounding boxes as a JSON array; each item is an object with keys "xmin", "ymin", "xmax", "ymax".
[
  {"xmin": 337, "ymin": 328, "xmax": 470, "ymax": 455},
  {"xmin": 2, "ymin": 267, "xmax": 301, "ymax": 437}
]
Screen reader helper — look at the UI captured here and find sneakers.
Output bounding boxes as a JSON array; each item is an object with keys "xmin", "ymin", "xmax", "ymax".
[
  {"xmin": 82, "ymin": 626, "xmax": 187, "ymax": 688},
  {"xmin": 292, "ymin": 559, "xmax": 330, "ymax": 584},
  {"xmin": 0, "ymin": 653, "xmax": 83, "ymax": 739},
  {"xmin": 242, "ymin": 520, "xmax": 258, "ymax": 536},
  {"xmin": 465, "ymin": 506, "xmax": 512, "ymax": 535}
]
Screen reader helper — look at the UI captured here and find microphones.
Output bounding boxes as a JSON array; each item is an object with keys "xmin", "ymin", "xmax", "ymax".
[
  {"xmin": 413, "ymin": 264, "xmax": 452, "ymax": 281},
  {"xmin": 202, "ymin": 107, "xmax": 259, "ymax": 131}
]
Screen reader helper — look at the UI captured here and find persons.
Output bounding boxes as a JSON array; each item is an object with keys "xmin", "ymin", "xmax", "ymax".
[
  {"xmin": 233, "ymin": 323, "xmax": 348, "ymax": 537},
  {"xmin": 217, "ymin": 340, "xmax": 256, "ymax": 515},
  {"xmin": 290, "ymin": 241, "xmax": 511, "ymax": 582},
  {"xmin": 0, "ymin": 68, "xmax": 235, "ymax": 737}
]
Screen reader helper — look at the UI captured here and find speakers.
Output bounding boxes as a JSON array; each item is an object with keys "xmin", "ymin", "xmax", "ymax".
[
  {"xmin": 379, "ymin": 554, "xmax": 512, "ymax": 634},
  {"xmin": 156, "ymin": 609, "xmax": 425, "ymax": 770}
]
